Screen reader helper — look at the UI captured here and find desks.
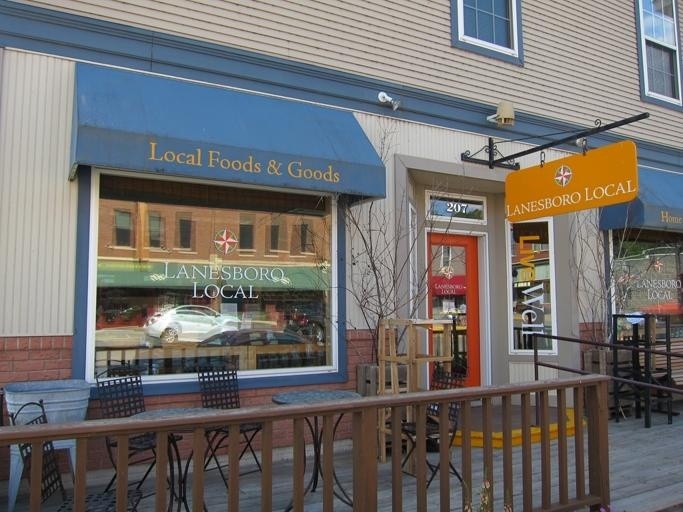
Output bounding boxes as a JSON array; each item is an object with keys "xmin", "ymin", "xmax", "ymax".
[
  {"xmin": 128, "ymin": 407, "xmax": 219, "ymax": 511},
  {"xmin": 271, "ymin": 388, "xmax": 361, "ymax": 511}
]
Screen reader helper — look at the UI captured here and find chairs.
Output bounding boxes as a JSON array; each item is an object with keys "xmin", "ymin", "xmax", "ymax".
[
  {"xmin": 394, "ymin": 366, "xmax": 467, "ymax": 490},
  {"xmin": 197, "ymin": 358, "xmax": 261, "ymax": 491},
  {"xmin": 7, "ymin": 399, "xmax": 142, "ymax": 511},
  {"xmin": 95, "ymin": 366, "xmax": 182, "ymax": 501}
]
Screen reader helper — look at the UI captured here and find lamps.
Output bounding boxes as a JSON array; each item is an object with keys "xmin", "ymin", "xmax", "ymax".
[
  {"xmin": 486, "ymin": 100, "xmax": 516, "ymax": 129},
  {"xmin": 377, "ymin": 90, "xmax": 402, "ymax": 113}
]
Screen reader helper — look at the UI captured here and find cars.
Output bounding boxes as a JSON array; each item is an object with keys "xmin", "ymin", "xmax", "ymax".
[{"xmin": 144, "ymin": 303, "xmax": 325, "ymax": 369}]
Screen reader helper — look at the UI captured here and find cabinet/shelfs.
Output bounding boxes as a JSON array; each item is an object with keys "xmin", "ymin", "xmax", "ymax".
[
  {"xmin": 611, "ymin": 313, "xmax": 674, "ymax": 429},
  {"xmin": 377, "ymin": 317, "xmax": 452, "ymax": 473}
]
